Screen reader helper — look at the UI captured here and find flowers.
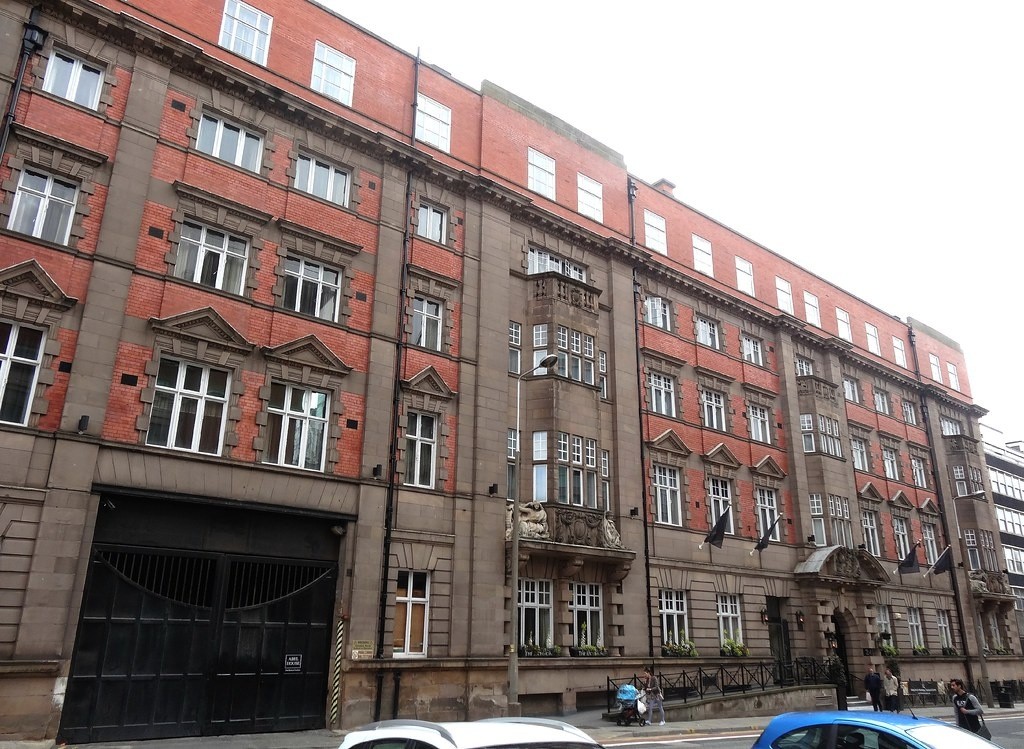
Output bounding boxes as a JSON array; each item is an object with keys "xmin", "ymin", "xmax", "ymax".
[{"xmin": 663, "ymin": 629, "xmax": 699, "ymax": 657}]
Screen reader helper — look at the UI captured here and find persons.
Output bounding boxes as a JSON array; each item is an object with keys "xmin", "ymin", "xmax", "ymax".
[
  {"xmin": 642, "ymin": 665, "xmax": 666, "ymax": 726},
  {"xmin": 863, "ymin": 668, "xmax": 884, "ymax": 712},
  {"xmin": 949, "ymin": 678, "xmax": 984, "ymax": 733},
  {"xmin": 883, "ymin": 668, "xmax": 899, "ymax": 713}
]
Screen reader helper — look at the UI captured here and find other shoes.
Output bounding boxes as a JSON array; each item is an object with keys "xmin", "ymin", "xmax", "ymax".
[
  {"xmin": 658, "ymin": 720, "xmax": 665, "ymax": 726},
  {"xmin": 645, "ymin": 720, "xmax": 652, "ymax": 726}
]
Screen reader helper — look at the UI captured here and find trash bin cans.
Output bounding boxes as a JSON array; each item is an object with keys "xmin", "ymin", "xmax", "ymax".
[{"xmin": 997, "ymin": 686, "xmax": 1014, "ymax": 708}]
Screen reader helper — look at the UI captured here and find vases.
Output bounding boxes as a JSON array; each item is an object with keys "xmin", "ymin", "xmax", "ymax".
[{"xmin": 661, "ymin": 648, "xmax": 690, "ymax": 657}]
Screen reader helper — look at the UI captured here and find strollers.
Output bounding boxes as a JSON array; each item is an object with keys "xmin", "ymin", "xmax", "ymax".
[{"xmin": 615, "ymin": 684, "xmax": 652, "ymax": 727}]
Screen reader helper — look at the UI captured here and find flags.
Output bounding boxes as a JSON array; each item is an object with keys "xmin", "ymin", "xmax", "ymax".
[
  {"xmin": 753, "ymin": 518, "xmax": 780, "ymax": 551},
  {"xmin": 704, "ymin": 509, "xmax": 729, "ymax": 549},
  {"xmin": 898, "ymin": 543, "xmax": 921, "ymax": 574},
  {"xmin": 933, "ymin": 547, "xmax": 953, "ymax": 575}
]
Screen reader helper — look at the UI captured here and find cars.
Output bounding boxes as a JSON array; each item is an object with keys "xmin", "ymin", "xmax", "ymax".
[
  {"xmin": 336, "ymin": 716, "xmax": 606, "ymax": 749},
  {"xmin": 747, "ymin": 709, "xmax": 1009, "ymax": 749}
]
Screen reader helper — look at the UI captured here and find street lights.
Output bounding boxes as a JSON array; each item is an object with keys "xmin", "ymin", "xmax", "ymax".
[{"xmin": 506, "ymin": 353, "xmax": 560, "ymax": 718}]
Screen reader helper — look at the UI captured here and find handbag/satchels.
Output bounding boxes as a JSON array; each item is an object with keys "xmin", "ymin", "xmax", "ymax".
[
  {"xmin": 975, "ymin": 725, "xmax": 992, "ymax": 739},
  {"xmin": 866, "ymin": 691, "xmax": 872, "ymax": 704}
]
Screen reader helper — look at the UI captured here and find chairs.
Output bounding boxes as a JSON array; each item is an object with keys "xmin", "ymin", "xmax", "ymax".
[{"xmin": 900, "ymin": 678, "xmax": 947, "ymax": 706}]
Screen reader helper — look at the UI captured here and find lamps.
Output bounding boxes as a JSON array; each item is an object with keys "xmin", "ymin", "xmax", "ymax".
[
  {"xmin": 78, "ymin": 415, "xmax": 89, "ymax": 431},
  {"xmin": 797, "ymin": 610, "xmax": 806, "ymax": 625},
  {"xmin": 808, "ymin": 534, "xmax": 815, "ymax": 543},
  {"xmin": 858, "ymin": 544, "xmax": 866, "ymax": 550},
  {"xmin": 629, "ymin": 507, "xmax": 638, "ymax": 517},
  {"xmin": 488, "ymin": 483, "xmax": 499, "ymax": 496},
  {"xmin": 958, "ymin": 562, "xmax": 964, "ymax": 567},
  {"xmin": 372, "ymin": 463, "xmax": 382, "ymax": 479},
  {"xmin": 875, "ymin": 629, "xmax": 891, "ymax": 642},
  {"xmin": 760, "ymin": 607, "xmax": 769, "ymax": 624},
  {"xmin": 825, "ymin": 631, "xmax": 838, "ymax": 649},
  {"xmin": 1003, "ymin": 568, "xmax": 1008, "ymax": 574}
]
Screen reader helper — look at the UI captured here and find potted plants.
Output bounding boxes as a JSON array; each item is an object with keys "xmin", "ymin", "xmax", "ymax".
[
  {"xmin": 721, "ymin": 629, "xmax": 751, "ymax": 657},
  {"xmin": 886, "ymin": 661, "xmax": 904, "ymax": 708},
  {"xmin": 568, "ymin": 621, "xmax": 608, "ymax": 657},
  {"xmin": 829, "ymin": 656, "xmax": 847, "ymax": 710},
  {"xmin": 520, "ymin": 631, "xmax": 560, "ymax": 657}
]
